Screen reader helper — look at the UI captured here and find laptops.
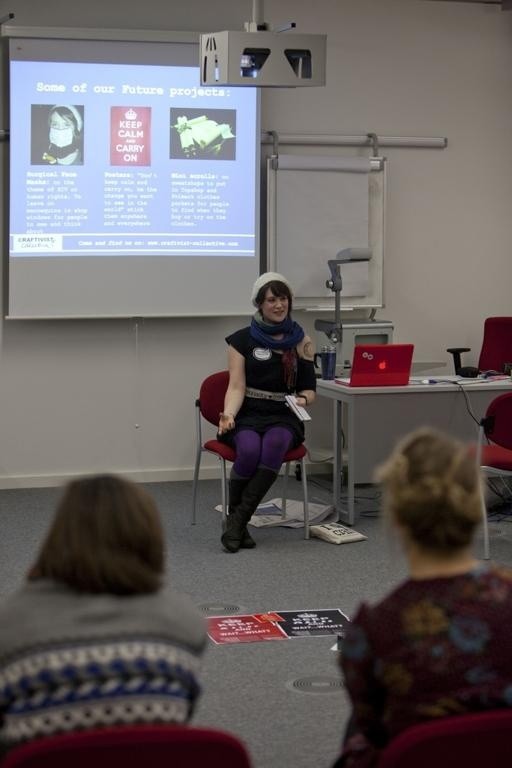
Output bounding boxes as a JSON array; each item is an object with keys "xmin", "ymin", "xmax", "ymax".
[{"xmin": 335, "ymin": 343, "xmax": 414, "ymax": 388}]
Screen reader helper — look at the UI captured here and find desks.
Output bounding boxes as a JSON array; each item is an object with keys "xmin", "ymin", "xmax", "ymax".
[{"xmin": 314, "ymin": 375, "xmax": 512, "ymax": 526}]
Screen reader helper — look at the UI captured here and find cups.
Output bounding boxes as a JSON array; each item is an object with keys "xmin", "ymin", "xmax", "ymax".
[{"xmin": 313, "ymin": 345, "xmax": 336, "ymax": 379}]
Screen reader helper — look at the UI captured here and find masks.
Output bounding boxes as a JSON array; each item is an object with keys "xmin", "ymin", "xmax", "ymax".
[{"xmin": 49, "ymin": 128, "xmax": 74, "ymax": 147}]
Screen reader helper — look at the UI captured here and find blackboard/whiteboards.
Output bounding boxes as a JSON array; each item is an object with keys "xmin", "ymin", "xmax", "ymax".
[{"xmin": 266, "ymin": 156, "xmax": 387, "ymax": 312}]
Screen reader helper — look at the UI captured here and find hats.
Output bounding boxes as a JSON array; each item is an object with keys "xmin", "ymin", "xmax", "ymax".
[
  {"xmin": 48, "ymin": 105, "xmax": 82, "ymax": 131},
  {"xmin": 251, "ymin": 272, "xmax": 294, "ymax": 308}
]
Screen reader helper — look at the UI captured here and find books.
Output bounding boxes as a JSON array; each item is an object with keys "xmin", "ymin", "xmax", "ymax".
[
  {"xmin": 285, "ymin": 393, "xmax": 311, "ymax": 423},
  {"xmin": 214, "ymin": 497, "xmax": 341, "ymax": 532}
]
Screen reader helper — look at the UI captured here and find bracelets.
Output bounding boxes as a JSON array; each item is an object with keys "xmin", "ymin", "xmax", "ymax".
[{"xmin": 227, "ymin": 413, "xmax": 235, "ymax": 417}]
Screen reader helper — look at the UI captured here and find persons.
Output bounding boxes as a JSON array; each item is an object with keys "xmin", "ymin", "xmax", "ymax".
[
  {"xmin": 337, "ymin": 427, "xmax": 510, "ymax": 767},
  {"xmin": 46, "ymin": 105, "xmax": 84, "ymax": 164},
  {"xmin": 1, "ymin": 472, "xmax": 206, "ymax": 748},
  {"xmin": 215, "ymin": 271, "xmax": 317, "ymax": 553}
]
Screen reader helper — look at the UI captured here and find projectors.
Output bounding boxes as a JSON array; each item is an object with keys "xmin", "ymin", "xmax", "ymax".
[{"xmin": 199, "ymin": 30, "xmax": 327, "ymax": 88}]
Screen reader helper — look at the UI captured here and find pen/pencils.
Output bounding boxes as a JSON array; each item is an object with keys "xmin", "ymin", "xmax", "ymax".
[{"xmin": 422, "ymin": 379, "xmax": 440, "ymax": 383}]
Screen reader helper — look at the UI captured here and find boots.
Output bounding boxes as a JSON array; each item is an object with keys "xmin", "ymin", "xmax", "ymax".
[{"xmin": 220, "ymin": 463, "xmax": 279, "ymax": 552}]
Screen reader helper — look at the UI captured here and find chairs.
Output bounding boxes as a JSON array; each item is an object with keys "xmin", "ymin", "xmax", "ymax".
[
  {"xmin": 469, "ymin": 391, "xmax": 512, "ymax": 559},
  {"xmin": 477, "ymin": 317, "xmax": 512, "ymax": 372},
  {"xmin": 379, "ymin": 708, "xmax": 512, "ymax": 767},
  {"xmin": 0, "ymin": 722, "xmax": 252, "ymax": 768},
  {"xmin": 190, "ymin": 370, "xmax": 311, "ymax": 540}
]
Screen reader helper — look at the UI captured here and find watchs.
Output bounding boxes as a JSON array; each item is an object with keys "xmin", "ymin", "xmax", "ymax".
[{"xmin": 299, "ymin": 395, "xmax": 308, "ymax": 403}]
